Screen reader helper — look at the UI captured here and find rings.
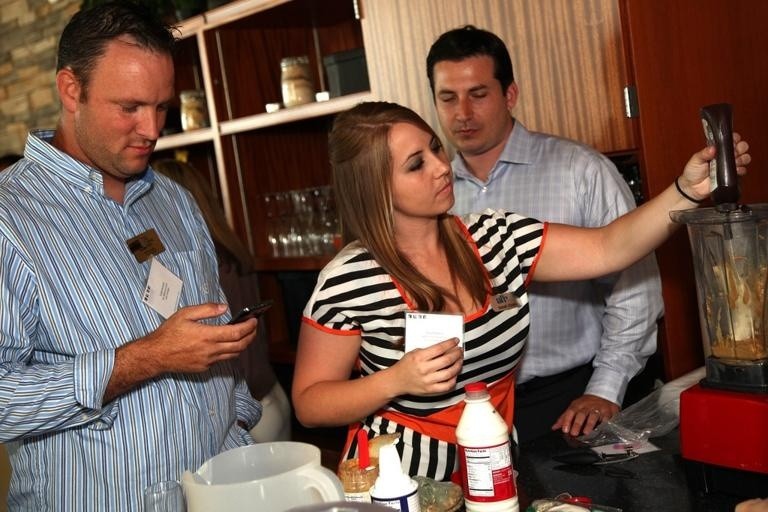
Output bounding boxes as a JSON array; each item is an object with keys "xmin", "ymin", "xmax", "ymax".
[{"xmin": 591, "ymin": 409, "xmax": 603, "ymax": 414}]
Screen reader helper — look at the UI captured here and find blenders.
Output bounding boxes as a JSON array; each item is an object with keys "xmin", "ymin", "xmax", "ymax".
[{"xmin": 669, "ymin": 102, "xmax": 767, "ymax": 474}]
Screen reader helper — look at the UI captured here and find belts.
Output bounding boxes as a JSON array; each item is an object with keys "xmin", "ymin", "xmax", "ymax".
[{"xmin": 516, "ymin": 376, "xmax": 567, "ymax": 398}]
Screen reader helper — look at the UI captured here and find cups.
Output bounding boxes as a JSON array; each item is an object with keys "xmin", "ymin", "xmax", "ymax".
[
  {"xmin": 143, "ymin": 480, "xmax": 184, "ymax": 512},
  {"xmin": 179, "ymin": 440, "xmax": 346, "ymax": 511}
]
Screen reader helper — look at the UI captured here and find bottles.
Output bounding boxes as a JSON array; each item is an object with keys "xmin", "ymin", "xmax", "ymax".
[
  {"xmin": 179, "ymin": 89, "xmax": 205, "ymax": 130},
  {"xmin": 454, "ymin": 382, "xmax": 520, "ymax": 512},
  {"xmin": 699, "ymin": 101, "xmax": 739, "ymax": 242},
  {"xmin": 366, "ymin": 444, "xmax": 421, "ymax": 512},
  {"xmin": 279, "ymin": 55, "xmax": 313, "ymax": 109}
]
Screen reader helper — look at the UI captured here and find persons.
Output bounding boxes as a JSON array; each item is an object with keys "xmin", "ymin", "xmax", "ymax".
[
  {"xmin": 0, "ymin": 0, "xmax": 258, "ymax": 512},
  {"xmin": 291, "ymin": 101, "xmax": 752, "ymax": 512},
  {"xmin": 426, "ymin": 24, "xmax": 665, "ymax": 438}
]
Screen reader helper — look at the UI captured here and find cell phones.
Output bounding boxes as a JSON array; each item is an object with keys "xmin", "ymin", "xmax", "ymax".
[{"xmin": 224, "ymin": 300, "xmax": 274, "ymax": 324}]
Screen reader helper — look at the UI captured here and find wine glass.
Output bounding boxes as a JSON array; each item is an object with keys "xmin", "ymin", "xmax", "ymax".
[{"xmin": 258, "ymin": 186, "xmax": 343, "ymax": 258}]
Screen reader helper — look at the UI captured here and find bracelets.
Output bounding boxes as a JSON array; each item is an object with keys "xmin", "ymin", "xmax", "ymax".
[{"xmin": 674, "ymin": 175, "xmax": 703, "ymax": 205}]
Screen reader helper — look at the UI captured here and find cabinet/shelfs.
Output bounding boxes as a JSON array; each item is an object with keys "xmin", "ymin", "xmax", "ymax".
[{"xmin": 150, "ymin": 0, "xmax": 376, "ymax": 273}]
